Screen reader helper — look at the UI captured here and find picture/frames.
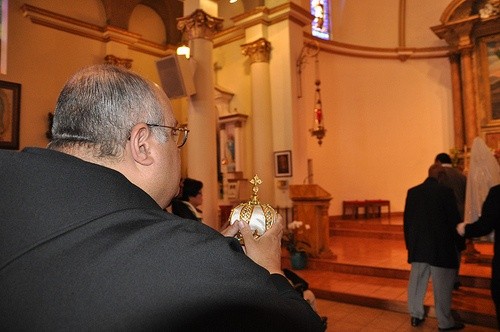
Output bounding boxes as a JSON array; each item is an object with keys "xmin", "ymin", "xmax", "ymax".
[
  {"xmin": 0, "ymin": 80, "xmax": 22, "ymax": 150},
  {"xmin": 274, "ymin": 150, "xmax": 291, "ymax": 177},
  {"xmin": 479, "ymin": 33, "xmax": 500, "ymax": 127}
]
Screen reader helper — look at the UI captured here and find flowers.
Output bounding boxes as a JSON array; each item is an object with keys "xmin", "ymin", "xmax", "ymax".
[{"xmin": 282, "ymin": 221, "xmax": 311, "ymax": 253}]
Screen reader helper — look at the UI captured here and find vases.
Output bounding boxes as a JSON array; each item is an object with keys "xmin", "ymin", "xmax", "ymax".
[{"xmin": 291, "ymin": 251, "xmax": 307, "ymax": 269}]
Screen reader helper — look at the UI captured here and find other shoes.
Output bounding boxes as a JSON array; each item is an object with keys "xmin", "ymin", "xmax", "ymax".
[
  {"xmin": 439, "ymin": 323, "xmax": 465, "ymax": 332},
  {"xmin": 411, "ymin": 317, "xmax": 425, "ymax": 327}
]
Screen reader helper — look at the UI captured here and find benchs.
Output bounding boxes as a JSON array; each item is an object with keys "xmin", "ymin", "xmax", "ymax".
[{"xmin": 343, "ymin": 199, "xmax": 390, "ymax": 219}]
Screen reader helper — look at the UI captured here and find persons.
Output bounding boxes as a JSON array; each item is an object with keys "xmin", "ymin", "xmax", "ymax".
[
  {"xmin": 171, "ymin": 178, "xmax": 203, "ymax": 222},
  {"xmin": 457, "ymin": 184, "xmax": 500, "ymax": 330},
  {"xmin": 403, "ymin": 152, "xmax": 466, "ymax": 332},
  {"xmin": 0, "ymin": 64, "xmax": 324, "ymax": 332}
]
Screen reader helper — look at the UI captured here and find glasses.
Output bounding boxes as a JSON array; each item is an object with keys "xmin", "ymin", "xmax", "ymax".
[{"xmin": 127, "ymin": 120, "xmax": 190, "ymax": 148}]
